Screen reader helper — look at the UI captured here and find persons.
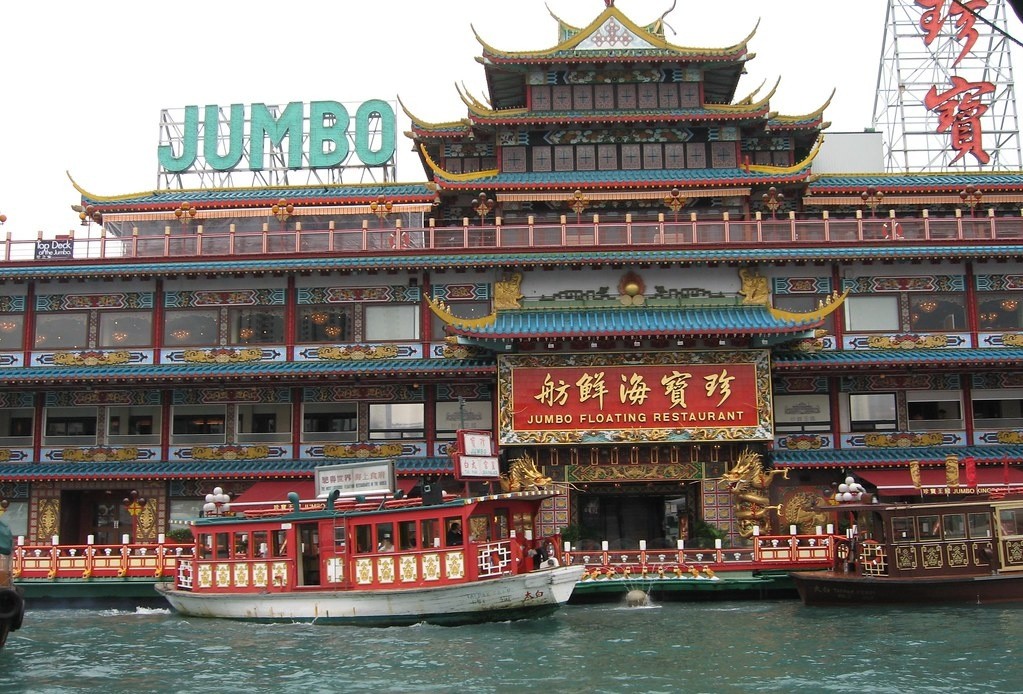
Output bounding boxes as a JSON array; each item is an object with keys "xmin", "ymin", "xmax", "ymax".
[
  {"xmin": 446, "ymin": 522, "xmax": 462, "ymax": 545},
  {"xmin": 377, "ymin": 531, "xmax": 394, "ymax": 552}
]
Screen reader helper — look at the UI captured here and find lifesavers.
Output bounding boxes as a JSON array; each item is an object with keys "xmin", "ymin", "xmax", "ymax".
[
  {"xmin": 543, "ymin": 537, "xmax": 558, "ymax": 557},
  {"xmin": 491, "ymin": 539, "xmax": 522, "ymax": 568},
  {"xmin": 833, "ymin": 540, "xmax": 852, "ymax": 563},
  {"xmin": 859, "ymin": 540, "xmax": 883, "ymax": 565}
]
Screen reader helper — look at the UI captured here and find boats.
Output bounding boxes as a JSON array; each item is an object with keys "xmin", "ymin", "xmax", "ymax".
[
  {"xmin": 787, "ymin": 498, "xmax": 1023, "ymax": 608},
  {"xmin": 154, "ymin": 489, "xmax": 588, "ymax": 629}
]
